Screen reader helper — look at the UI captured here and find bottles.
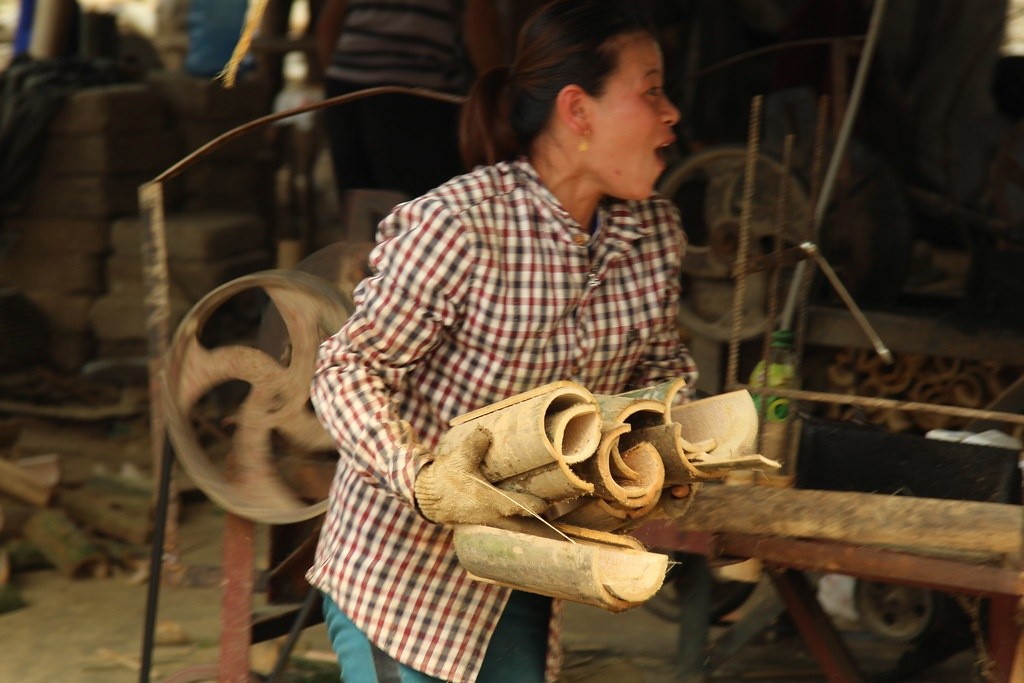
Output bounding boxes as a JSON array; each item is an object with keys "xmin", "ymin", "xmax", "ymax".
[{"xmin": 742, "ymin": 330, "xmax": 798, "ymax": 420}]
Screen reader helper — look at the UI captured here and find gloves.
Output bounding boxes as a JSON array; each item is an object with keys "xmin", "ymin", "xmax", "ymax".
[{"xmin": 414, "ymin": 427, "xmax": 548, "ymax": 532}]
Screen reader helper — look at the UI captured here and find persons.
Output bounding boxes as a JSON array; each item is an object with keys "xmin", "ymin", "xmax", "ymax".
[
  {"xmin": 183, "ymin": 0, "xmax": 294, "ymax": 102},
  {"xmin": 312, "ymin": 0, "xmax": 508, "ymax": 244},
  {"xmin": 304, "ymin": 0, "xmax": 699, "ymax": 683}
]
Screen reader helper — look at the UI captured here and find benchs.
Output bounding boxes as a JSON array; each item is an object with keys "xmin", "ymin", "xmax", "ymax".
[{"xmin": 610, "ymin": 484, "xmax": 1024, "ymax": 683}]
